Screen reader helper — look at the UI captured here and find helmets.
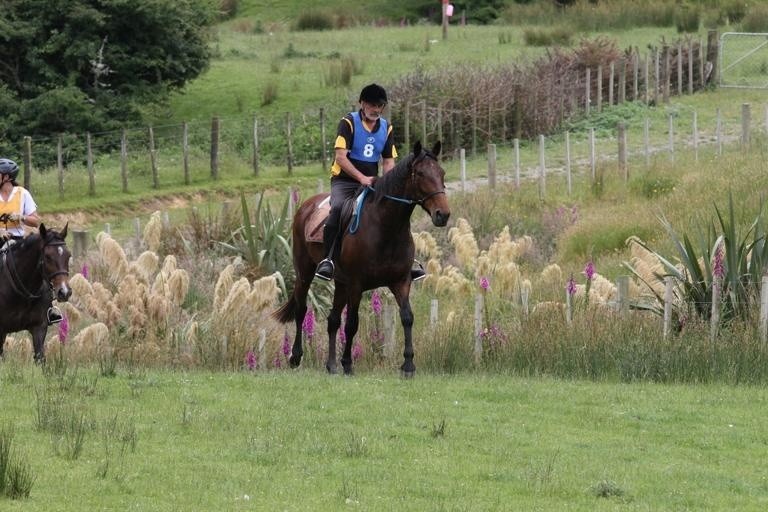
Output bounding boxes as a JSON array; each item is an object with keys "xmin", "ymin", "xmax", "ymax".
[
  {"xmin": 0, "ymin": 158, "xmax": 20, "ymax": 181},
  {"xmin": 359, "ymin": 83, "xmax": 388, "ymax": 105}
]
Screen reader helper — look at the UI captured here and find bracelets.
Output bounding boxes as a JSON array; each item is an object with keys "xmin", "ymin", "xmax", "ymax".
[{"xmin": 22, "ymin": 216, "xmax": 25, "ymax": 224}]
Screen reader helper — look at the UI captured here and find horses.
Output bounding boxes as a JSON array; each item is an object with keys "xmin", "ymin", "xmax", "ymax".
[
  {"xmin": 270, "ymin": 138, "xmax": 451, "ymax": 382},
  {"xmin": 0, "ymin": 222, "xmax": 72, "ymax": 374}
]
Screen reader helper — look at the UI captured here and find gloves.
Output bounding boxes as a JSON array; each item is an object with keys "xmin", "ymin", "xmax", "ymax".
[{"xmin": 8, "ymin": 213, "xmax": 25, "ymax": 222}]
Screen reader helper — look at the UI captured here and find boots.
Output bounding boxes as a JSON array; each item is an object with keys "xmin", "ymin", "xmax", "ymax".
[
  {"xmin": 315, "ymin": 222, "xmax": 343, "ymax": 281},
  {"xmin": 411, "ymin": 258, "xmax": 426, "ymax": 282},
  {"xmin": 50, "ymin": 292, "xmax": 63, "ymax": 323}
]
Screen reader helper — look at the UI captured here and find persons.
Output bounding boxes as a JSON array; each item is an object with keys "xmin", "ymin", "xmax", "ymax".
[
  {"xmin": 318, "ymin": 83, "xmax": 423, "ymax": 278},
  {"xmin": 0, "ymin": 158, "xmax": 63, "ymax": 324}
]
